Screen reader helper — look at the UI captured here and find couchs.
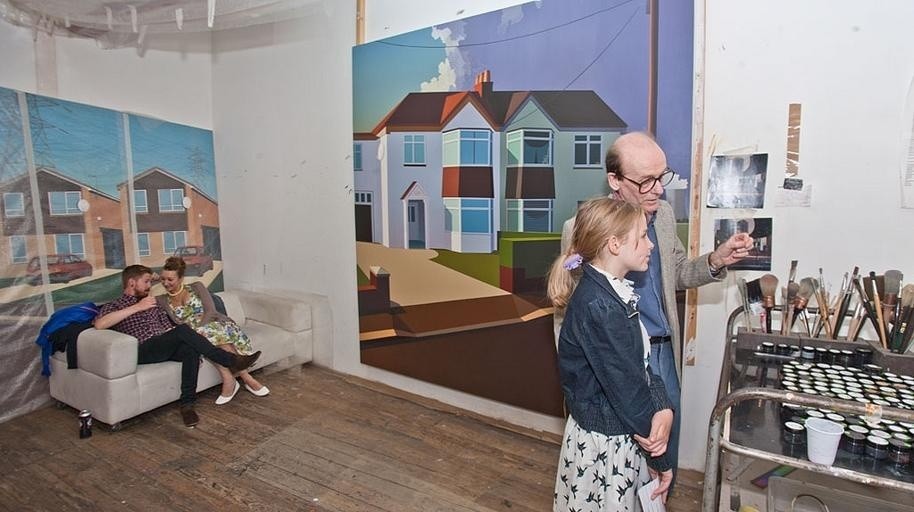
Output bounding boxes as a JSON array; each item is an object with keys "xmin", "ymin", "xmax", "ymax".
[{"xmin": 46, "ymin": 283, "xmax": 314, "ymax": 433}]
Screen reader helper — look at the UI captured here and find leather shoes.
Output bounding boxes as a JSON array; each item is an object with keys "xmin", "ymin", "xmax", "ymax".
[
  {"xmin": 214, "ymin": 381, "xmax": 240, "ymax": 405},
  {"xmin": 244, "ymin": 382, "xmax": 270, "ymax": 397}
]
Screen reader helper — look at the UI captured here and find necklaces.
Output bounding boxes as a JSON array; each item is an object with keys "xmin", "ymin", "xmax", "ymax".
[{"xmin": 167, "ymin": 283, "xmax": 184, "ymax": 297}]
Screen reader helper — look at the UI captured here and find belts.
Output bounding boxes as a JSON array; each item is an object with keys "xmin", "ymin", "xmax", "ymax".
[{"xmin": 648, "ymin": 335, "xmax": 670, "ymax": 344}]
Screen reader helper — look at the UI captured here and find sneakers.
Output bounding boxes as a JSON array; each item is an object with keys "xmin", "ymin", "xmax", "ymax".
[
  {"xmin": 229, "ymin": 351, "xmax": 262, "ymax": 373},
  {"xmin": 181, "ymin": 406, "xmax": 199, "ymax": 426}
]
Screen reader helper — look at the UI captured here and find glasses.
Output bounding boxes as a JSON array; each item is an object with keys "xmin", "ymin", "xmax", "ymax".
[{"xmin": 615, "ymin": 167, "xmax": 675, "ymax": 194}]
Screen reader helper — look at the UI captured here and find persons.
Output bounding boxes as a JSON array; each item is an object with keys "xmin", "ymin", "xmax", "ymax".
[
  {"xmin": 547, "ymin": 196, "xmax": 677, "ymax": 512},
  {"xmin": 553, "ymin": 129, "xmax": 756, "ymax": 504},
  {"xmin": 149, "ymin": 256, "xmax": 269, "ymax": 406},
  {"xmin": 91, "ymin": 264, "xmax": 262, "ymax": 427}
]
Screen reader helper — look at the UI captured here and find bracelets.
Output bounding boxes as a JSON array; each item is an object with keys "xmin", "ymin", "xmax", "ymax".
[{"xmin": 709, "ymin": 252, "xmax": 721, "ymax": 277}]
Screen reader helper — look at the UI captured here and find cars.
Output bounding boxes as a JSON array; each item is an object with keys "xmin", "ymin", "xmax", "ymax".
[
  {"xmin": 23, "ymin": 252, "xmax": 94, "ymax": 286},
  {"xmin": 171, "ymin": 245, "xmax": 215, "ymax": 279}
]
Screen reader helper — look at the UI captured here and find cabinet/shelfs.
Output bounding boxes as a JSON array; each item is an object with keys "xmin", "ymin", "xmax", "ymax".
[{"xmin": 698, "ymin": 302, "xmax": 914, "ymax": 512}]
